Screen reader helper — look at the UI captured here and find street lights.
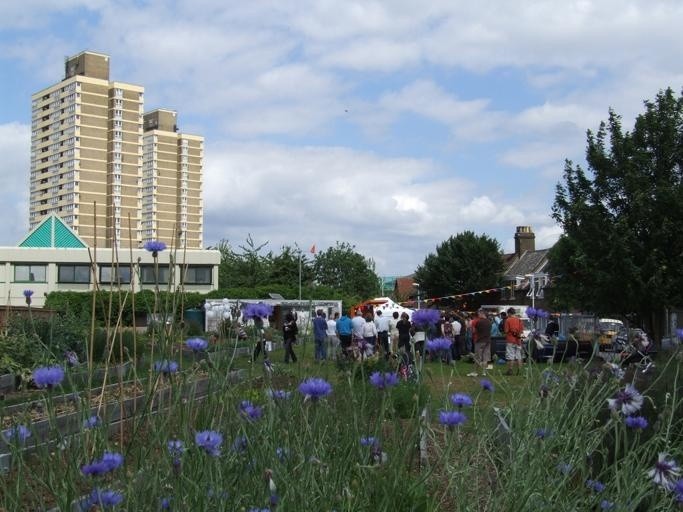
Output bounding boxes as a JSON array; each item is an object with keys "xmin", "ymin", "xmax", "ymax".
[
  {"xmin": 525, "ymin": 273, "xmax": 535, "ymax": 310},
  {"xmin": 411, "ymin": 282, "xmax": 420, "ymax": 310}
]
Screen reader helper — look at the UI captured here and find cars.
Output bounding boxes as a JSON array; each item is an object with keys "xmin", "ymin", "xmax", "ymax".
[{"xmin": 465, "ymin": 304, "xmax": 650, "ymax": 362}]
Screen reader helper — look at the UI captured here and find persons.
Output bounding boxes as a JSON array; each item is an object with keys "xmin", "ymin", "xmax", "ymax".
[{"xmin": 238, "ymin": 324, "xmax": 246, "ymax": 341}]
